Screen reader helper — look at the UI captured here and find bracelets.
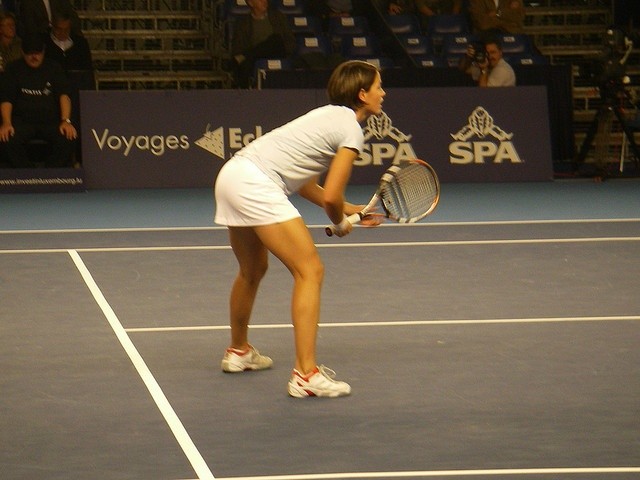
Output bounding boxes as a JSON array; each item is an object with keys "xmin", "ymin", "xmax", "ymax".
[{"xmin": 333, "ymin": 213, "xmax": 348, "ymax": 232}]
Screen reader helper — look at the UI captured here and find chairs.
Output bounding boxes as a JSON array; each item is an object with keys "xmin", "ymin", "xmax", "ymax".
[
  {"xmin": 388, "ymin": 16, "xmax": 478, "ymax": 74},
  {"xmin": 342, "ymin": 34, "xmax": 385, "ymax": 57},
  {"xmin": 329, "ymin": 15, "xmax": 368, "ymax": 35},
  {"xmin": 295, "ymin": 35, "xmax": 332, "ymax": 55},
  {"xmin": 497, "ymin": 32, "xmax": 531, "ymax": 56},
  {"xmin": 353, "ymin": 56, "xmax": 393, "ymax": 87},
  {"xmin": 276, "ymin": 0, "xmax": 304, "ymax": 15},
  {"xmin": 287, "ymin": 16, "xmax": 323, "ymax": 33},
  {"xmin": 505, "ymin": 54, "xmax": 534, "ymax": 66}
]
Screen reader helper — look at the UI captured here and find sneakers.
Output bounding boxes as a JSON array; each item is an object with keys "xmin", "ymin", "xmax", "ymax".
[
  {"xmin": 221, "ymin": 344, "xmax": 273, "ymax": 373},
  {"xmin": 287, "ymin": 364, "xmax": 351, "ymax": 398}
]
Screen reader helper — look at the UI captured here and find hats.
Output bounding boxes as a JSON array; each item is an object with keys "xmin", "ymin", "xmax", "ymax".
[{"xmin": 21, "ymin": 32, "xmax": 44, "ymax": 53}]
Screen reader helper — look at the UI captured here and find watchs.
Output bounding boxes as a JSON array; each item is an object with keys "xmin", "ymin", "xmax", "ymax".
[{"xmin": 60, "ymin": 119, "xmax": 71, "ymax": 124}]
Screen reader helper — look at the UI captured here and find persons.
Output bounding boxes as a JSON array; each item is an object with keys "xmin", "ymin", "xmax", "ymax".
[
  {"xmin": 382, "ymin": 0, "xmax": 412, "ymax": 15},
  {"xmin": 0, "ymin": 32, "xmax": 80, "ymax": 168},
  {"xmin": 44, "ymin": 13, "xmax": 96, "ymax": 90},
  {"xmin": 214, "ymin": 60, "xmax": 385, "ymax": 398},
  {"xmin": 0, "ymin": 13, "xmax": 22, "ymax": 71},
  {"xmin": 457, "ymin": 38, "xmax": 516, "ymax": 87},
  {"xmin": 468, "ymin": 0, "xmax": 528, "ymax": 44},
  {"xmin": 417, "ymin": 0, "xmax": 465, "ymax": 20},
  {"xmin": 319, "ymin": 1, "xmax": 352, "ymax": 40},
  {"xmin": 14, "ymin": 1, "xmax": 78, "ymax": 47},
  {"xmin": 232, "ymin": 0, "xmax": 297, "ymax": 91}
]
